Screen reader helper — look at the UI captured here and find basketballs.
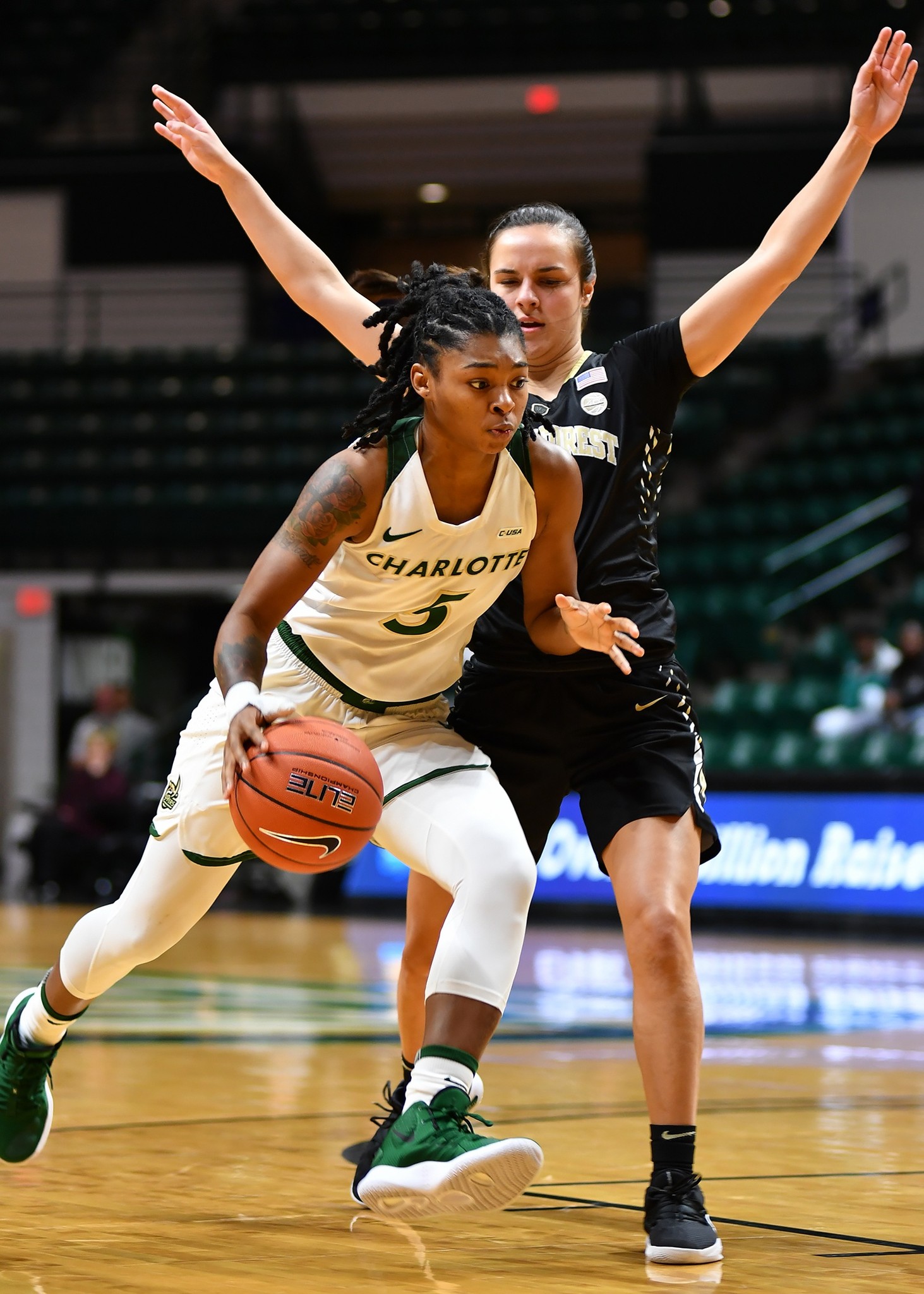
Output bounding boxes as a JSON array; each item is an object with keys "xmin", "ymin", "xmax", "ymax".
[{"xmin": 229, "ymin": 713, "xmax": 386, "ymax": 875}]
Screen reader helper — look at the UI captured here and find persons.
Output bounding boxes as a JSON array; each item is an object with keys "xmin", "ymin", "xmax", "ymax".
[
  {"xmin": 689, "ymin": 602, "xmax": 924, "ymax": 764},
  {"xmin": 151, "ymin": 27, "xmax": 918, "ymax": 1264},
  {"xmin": 0, "ymin": 266, "xmax": 644, "ymax": 1227},
  {"xmin": 26, "ymin": 681, "xmax": 164, "ymax": 903}
]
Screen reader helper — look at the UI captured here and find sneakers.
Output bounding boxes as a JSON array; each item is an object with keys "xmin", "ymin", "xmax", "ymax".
[
  {"xmin": 643, "ymin": 1170, "xmax": 722, "ymax": 1264},
  {"xmin": 351, "ymin": 1080, "xmax": 411, "ymax": 1205},
  {"xmin": 358, "ymin": 1086, "xmax": 543, "ymax": 1220},
  {"xmin": 0, "ymin": 987, "xmax": 68, "ymax": 1165}
]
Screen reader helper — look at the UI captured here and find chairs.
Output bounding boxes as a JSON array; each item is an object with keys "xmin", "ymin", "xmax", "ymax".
[{"xmin": 0, "ymin": 337, "xmax": 924, "ymax": 798}]
